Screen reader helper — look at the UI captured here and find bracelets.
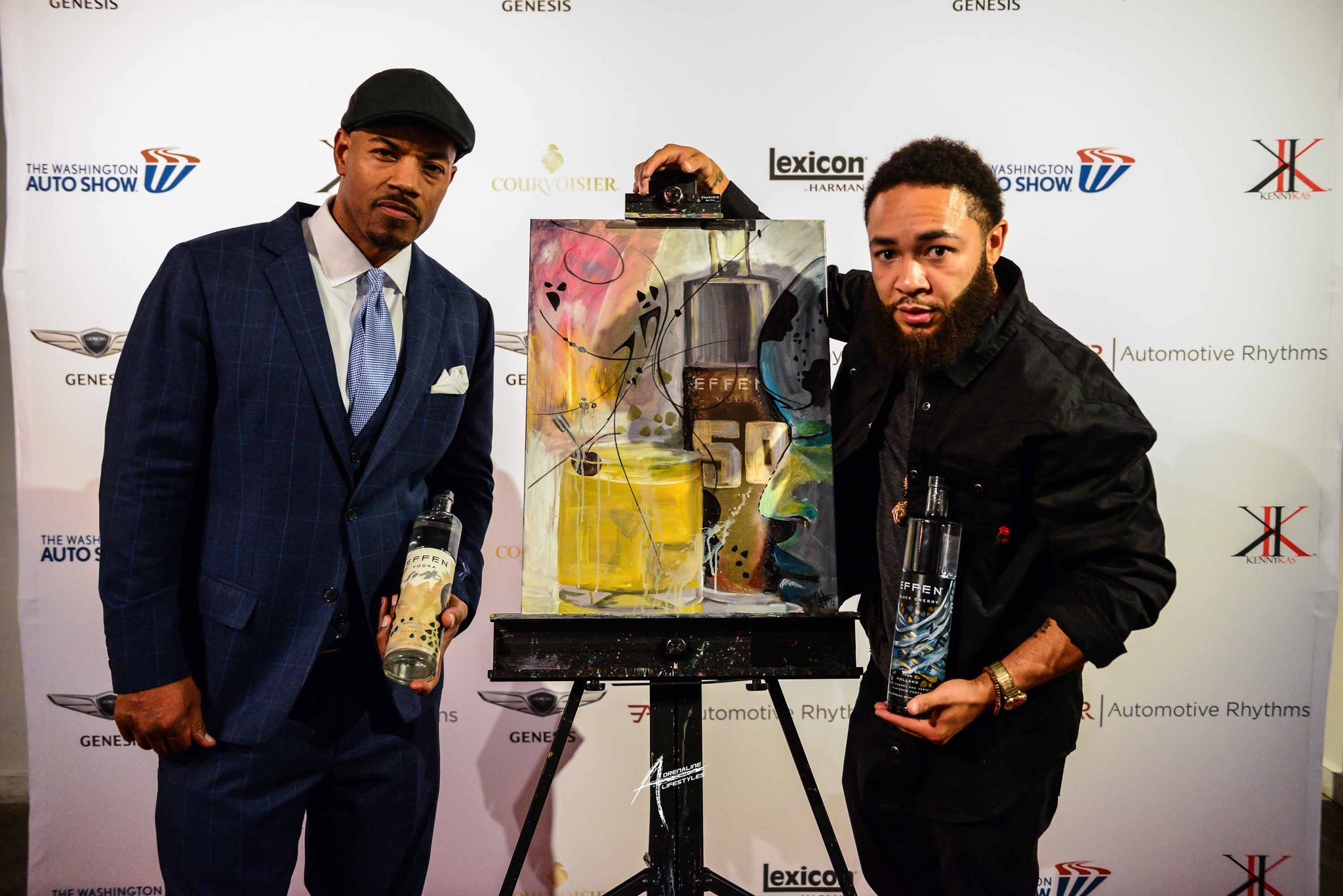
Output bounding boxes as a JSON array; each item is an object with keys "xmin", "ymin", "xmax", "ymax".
[{"xmin": 982, "ymin": 666, "xmax": 1002, "ymax": 715}]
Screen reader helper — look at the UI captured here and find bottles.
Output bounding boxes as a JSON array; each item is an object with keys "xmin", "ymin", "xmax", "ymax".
[
  {"xmin": 887, "ymin": 475, "xmax": 963, "ymax": 718},
  {"xmin": 384, "ymin": 490, "xmax": 461, "ymax": 685}
]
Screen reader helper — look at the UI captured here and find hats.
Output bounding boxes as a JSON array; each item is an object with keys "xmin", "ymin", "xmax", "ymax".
[{"xmin": 340, "ymin": 68, "xmax": 476, "ymax": 166}]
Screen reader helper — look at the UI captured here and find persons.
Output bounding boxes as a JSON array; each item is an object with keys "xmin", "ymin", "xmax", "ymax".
[
  {"xmin": 634, "ymin": 138, "xmax": 1177, "ymax": 894},
  {"xmin": 96, "ymin": 68, "xmax": 497, "ymax": 896}
]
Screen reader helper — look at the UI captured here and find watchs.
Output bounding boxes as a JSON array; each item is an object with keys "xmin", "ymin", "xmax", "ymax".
[{"xmin": 989, "ymin": 662, "xmax": 1026, "ymax": 711}]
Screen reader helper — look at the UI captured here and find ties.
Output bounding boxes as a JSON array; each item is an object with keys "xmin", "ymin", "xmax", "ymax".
[{"xmin": 347, "ymin": 268, "xmax": 398, "ymax": 438}]
